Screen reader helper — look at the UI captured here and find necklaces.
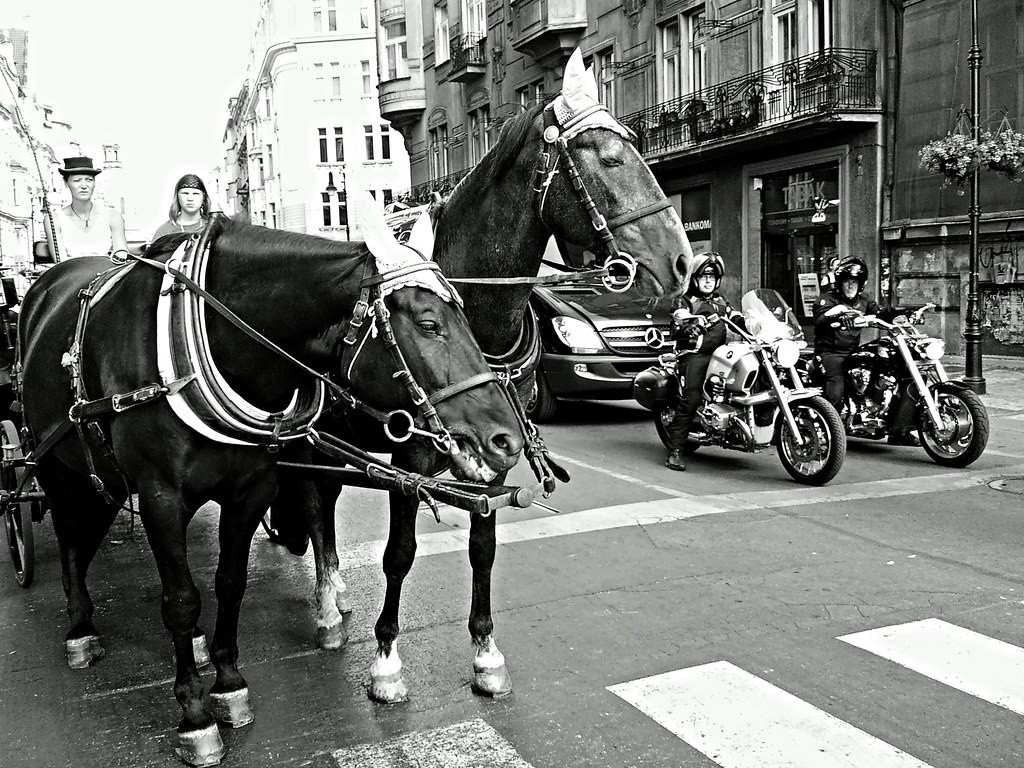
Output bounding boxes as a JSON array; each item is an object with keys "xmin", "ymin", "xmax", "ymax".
[{"xmin": 70, "ymin": 201, "xmax": 93, "ymax": 227}]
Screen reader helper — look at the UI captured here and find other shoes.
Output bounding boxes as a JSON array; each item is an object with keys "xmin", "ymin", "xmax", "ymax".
[
  {"xmin": 664, "ymin": 448, "xmax": 686, "ymax": 470},
  {"xmin": 887, "ymin": 433, "xmax": 923, "ymax": 447}
]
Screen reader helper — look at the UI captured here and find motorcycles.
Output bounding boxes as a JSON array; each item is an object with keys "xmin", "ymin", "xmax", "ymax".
[
  {"xmin": 776, "ymin": 301, "xmax": 989, "ymax": 468},
  {"xmin": 633, "ymin": 288, "xmax": 847, "ymax": 486}
]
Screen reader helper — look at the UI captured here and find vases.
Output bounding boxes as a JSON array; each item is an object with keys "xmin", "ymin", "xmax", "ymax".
[
  {"xmin": 988, "ymin": 160, "xmax": 1007, "ymax": 172},
  {"xmin": 939, "ymin": 162, "xmax": 957, "ymax": 177}
]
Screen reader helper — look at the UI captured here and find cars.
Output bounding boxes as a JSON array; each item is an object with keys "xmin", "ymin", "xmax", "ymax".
[{"xmin": 383, "ymin": 204, "xmax": 675, "ymax": 425}]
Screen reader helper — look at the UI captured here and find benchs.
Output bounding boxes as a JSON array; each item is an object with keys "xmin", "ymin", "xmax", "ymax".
[{"xmin": 32, "ymin": 240, "xmax": 145, "ymax": 275}]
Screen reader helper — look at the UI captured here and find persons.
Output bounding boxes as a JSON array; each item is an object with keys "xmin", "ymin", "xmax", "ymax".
[
  {"xmin": 662, "ymin": 250, "xmax": 745, "ymax": 473},
  {"xmin": 152, "ymin": 173, "xmax": 211, "ymax": 241},
  {"xmin": 813, "ymin": 255, "xmax": 926, "ymax": 415},
  {"xmin": 821, "ymin": 256, "xmax": 842, "ymax": 291},
  {"xmin": 43, "ymin": 157, "xmax": 130, "ymax": 264}
]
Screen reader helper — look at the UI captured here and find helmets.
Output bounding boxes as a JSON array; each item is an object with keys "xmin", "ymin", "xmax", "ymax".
[
  {"xmin": 691, "ymin": 251, "xmax": 725, "ymax": 291},
  {"xmin": 834, "ymin": 262, "xmax": 869, "ymax": 292}
]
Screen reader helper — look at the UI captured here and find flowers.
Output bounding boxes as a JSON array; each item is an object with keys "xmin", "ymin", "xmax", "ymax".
[
  {"xmin": 917, "ymin": 132, "xmax": 992, "ymax": 194},
  {"xmin": 983, "ymin": 130, "xmax": 1024, "ymax": 183}
]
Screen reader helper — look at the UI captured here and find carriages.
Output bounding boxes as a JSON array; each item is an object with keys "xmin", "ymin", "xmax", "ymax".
[{"xmin": 0, "ymin": 46, "xmax": 693, "ymax": 767}]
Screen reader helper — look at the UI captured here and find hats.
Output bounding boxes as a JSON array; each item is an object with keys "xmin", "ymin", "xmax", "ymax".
[{"xmin": 59, "ymin": 157, "xmax": 102, "ymax": 176}]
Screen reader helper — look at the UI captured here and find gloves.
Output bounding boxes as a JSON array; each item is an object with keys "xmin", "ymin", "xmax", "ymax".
[
  {"xmin": 836, "ymin": 312, "xmax": 855, "ymax": 332},
  {"xmin": 683, "ymin": 324, "xmax": 707, "ymax": 340},
  {"xmin": 908, "ymin": 311, "xmax": 926, "ymax": 326}
]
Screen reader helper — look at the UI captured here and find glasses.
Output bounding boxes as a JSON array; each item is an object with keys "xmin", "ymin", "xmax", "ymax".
[
  {"xmin": 698, "ymin": 275, "xmax": 714, "ymax": 281},
  {"xmin": 837, "ymin": 255, "xmax": 869, "ymax": 273}
]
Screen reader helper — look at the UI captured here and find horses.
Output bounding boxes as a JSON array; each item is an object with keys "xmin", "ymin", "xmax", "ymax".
[{"xmin": 17, "ymin": 45, "xmax": 696, "ymax": 768}]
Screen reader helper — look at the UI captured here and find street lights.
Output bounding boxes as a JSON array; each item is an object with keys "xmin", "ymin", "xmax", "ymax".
[
  {"xmin": 325, "ymin": 164, "xmax": 349, "ymax": 241},
  {"xmin": 30, "ymin": 191, "xmax": 48, "ymax": 243}
]
